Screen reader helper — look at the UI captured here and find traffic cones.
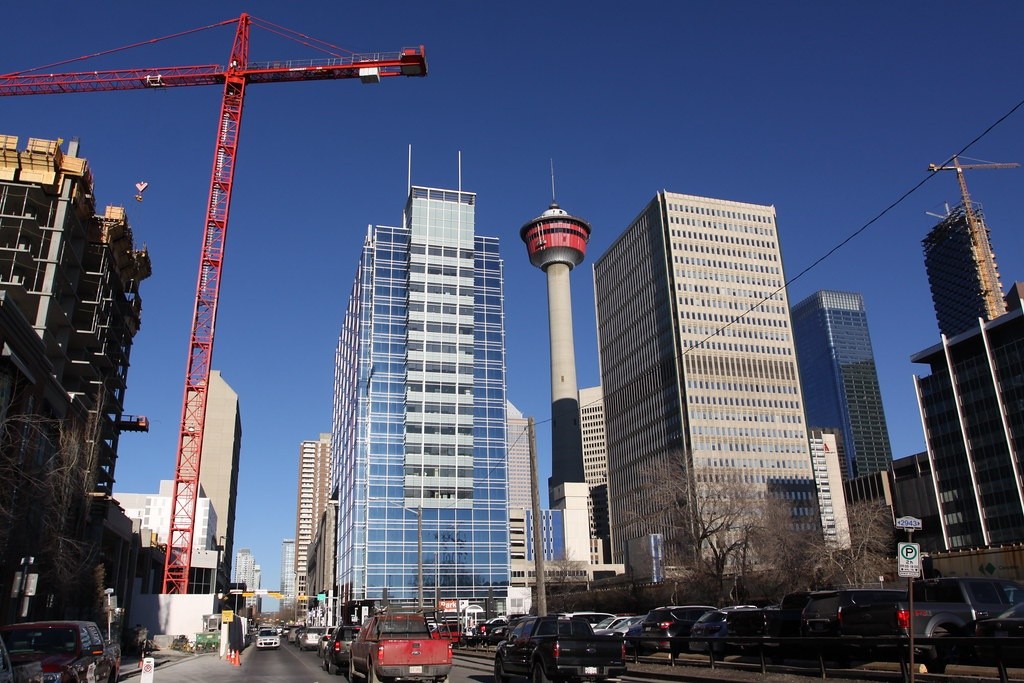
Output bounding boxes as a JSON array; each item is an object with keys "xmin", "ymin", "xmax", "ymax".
[
  {"xmin": 226, "ymin": 648, "xmax": 231, "ymax": 661},
  {"xmin": 230, "ymin": 650, "xmax": 235, "ymax": 665},
  {"xmin": 234, "ymin": 650, "xmax": 242, "ymax": 667}
]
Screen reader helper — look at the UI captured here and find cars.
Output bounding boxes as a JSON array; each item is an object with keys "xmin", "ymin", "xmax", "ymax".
[
  {"xmin": 975, "ymin": 599, "xmax": 1024, "ymax": 665},
  {"xmin": 428, "ymin": 612, "xmax": 533, "ymax": 648},
  {"xmin": 258, "ymin": 624, "xmax": 301, "ymax": 645}
]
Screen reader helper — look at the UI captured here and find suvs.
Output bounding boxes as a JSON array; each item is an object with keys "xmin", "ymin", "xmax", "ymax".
[
  {"xmin": 255, "ymin": 628, "xmax": 282, "ymax": 650},
  {"xmin": 299, "ymin": 626, "xmax": 326, "ymax": 652},
  {"xmin": 549, "ymin": 589, "xmax": 813, "ymax": 657},
  {"xmin": 322, "ymin": 623, "xmax": 364, "ymax": 675},
  {"xmin": 317, "ymin": 626, "xmax": 337, "ymax": 658},
  {"xmin": 799, "ymin": 588, "xmax": 907, "ymax": 667}
]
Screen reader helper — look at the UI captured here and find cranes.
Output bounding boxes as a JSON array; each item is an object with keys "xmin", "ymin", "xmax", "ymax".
[
  {"xmin": 0, "ymin": 11, "xmax": 429, "ymax": 595},
  {"xmin": 928, "ymin": 154, "xmax": 1022, "ymax": 207}
]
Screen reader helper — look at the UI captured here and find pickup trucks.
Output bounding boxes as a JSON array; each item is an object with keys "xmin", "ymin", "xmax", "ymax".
[
  {"xmin": 837, "ymin": 576, "xmax": 1024, "ymax": 675},
  {"xmin": 1, "ymin": 620, "xmax": 122, "ymax": 683},
  {"xmin": 349, "ymin": 612, "xmax": 453, "ymax": 683},
  {"xmin": 494, "ymin": 616, "xmax": 628, "ymax": 683}
]
